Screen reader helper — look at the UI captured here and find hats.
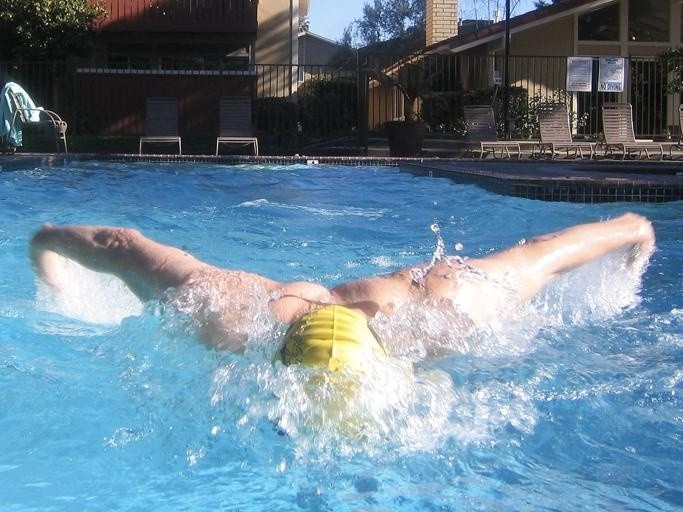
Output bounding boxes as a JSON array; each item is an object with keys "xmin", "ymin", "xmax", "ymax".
[{"xmin": 281, "ymin": 305, "xmax": 415, "ymax": 441}]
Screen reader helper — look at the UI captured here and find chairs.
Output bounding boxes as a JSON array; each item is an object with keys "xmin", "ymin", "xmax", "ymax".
[
  {"xmin": 0, "ymin": 91, "xmax": 68, "ymax": 153},
  {"xmin": 216, "ymin": 96, "xmax": 258, "ymax": 156},
  {"xmin": 535, "ymin": 102, "xmax": 593, "ymax": 160},
  {"xmin": 463, "ymin": 105, "xmax": 521, "ymax": 160},
  {"xmin": 669, "ymin": 103, "xmax": 683, "ymax": 158},
  {"xmin": 139, "ymin": 97, "xmax": 181, "ymax": 155},
  {"xmin": 602, "ymin": 102, "xmax": 663, "ymax": 161}
]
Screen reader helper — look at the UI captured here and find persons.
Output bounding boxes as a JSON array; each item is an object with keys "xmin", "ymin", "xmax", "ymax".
[{"xmin": 28, "ymin": 205, "xmax": 655, "ymax": 431}]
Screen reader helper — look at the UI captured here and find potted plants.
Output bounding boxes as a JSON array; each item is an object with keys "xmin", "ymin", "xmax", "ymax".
[{"xmin": 353, "ymin": 49, "xmax": 465, "ymax": 157}]
